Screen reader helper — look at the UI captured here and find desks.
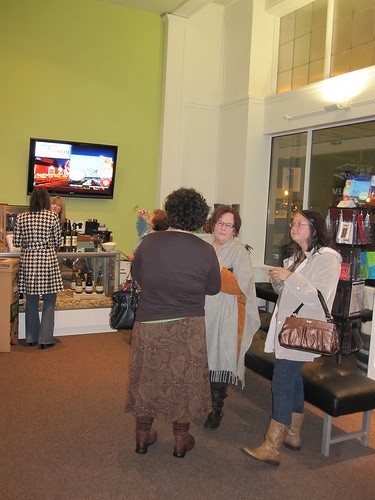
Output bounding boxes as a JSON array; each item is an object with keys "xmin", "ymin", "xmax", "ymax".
[{"xmin": 0, "ymin": 259, "xmax": 20, "ymax": 353}]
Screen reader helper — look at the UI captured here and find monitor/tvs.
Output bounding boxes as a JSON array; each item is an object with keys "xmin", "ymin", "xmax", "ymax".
[{"xmin": 28, "ymin": 137, "xmax": 118, "ymax": 200}]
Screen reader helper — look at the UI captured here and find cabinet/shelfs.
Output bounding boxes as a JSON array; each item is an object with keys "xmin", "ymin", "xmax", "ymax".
[{"xmin": 330, "ymin": 209, "xmax": 375, "ymax": 366}]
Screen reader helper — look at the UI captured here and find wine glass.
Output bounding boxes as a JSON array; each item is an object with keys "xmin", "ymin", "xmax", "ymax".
[{"xmin": 133, "ymin": 205, "xmax": 151, "ymax": 220}]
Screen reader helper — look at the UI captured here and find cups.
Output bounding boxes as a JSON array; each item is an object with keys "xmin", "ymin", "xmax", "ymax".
[{"xmin": 93, "ymin": 234, "xmax": 104, "ymax": 252}]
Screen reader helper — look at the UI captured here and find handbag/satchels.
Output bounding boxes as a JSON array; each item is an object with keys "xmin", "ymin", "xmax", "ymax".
[
  {"xmin": 278, "ymin": 289, "xmax": 340, "ymax": 356},
  {"xmin": 110, "ymin": 278, "xmax": 140, "ymax": 329}
]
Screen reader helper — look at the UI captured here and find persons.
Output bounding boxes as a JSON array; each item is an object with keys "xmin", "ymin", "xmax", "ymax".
[
  {"xmin": 127, "ymin": 208, "xmax": 169, "ymax": 260},
  {"xmin": 12, "ymin": 186, "xmax": 63, "ymax": 348},
  {"xmin": 51, "ymin": 196, "xmax": 65, "ymax": 222},
  {"xmin": 125, "ymin": 189, "xmax": 221, "ymax": 457},
  {"xmin": 242, "ymin": 210, "xmax": 342, "ymax": 463},
  {"xmin": 203, "ymin": 208, "xmax": 259, "ymax": 429}
]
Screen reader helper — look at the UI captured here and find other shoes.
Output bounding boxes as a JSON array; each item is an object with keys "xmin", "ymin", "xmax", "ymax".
[
  {"xmin": 29, "ymin": 342, "xmax": 38, "ymax": 346},
  {"xmin": 41, "ymin": 344, "xmax": 55, "ymax": 349}
]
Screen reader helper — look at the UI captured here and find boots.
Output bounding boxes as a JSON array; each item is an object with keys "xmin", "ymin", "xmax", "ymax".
[
  {"xmin": 135, "ymin": 416, "xmax": 152, "ymax": 453},
  {"xmin": 284, "ymin": 412, "xmax": 305, "ymax": 450},
  {"xmin": 172, "ymin": 422, "xmax": 195, "ymax": 457},
  {"xmin": 241, "ymin": 418, "xmax": 289, "ymax": 465},
  {"xmin": 204, "ymin": 391, "xmax": 224, "ymax": 428}
]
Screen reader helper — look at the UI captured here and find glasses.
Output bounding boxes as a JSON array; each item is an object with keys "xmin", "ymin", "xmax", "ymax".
[
  {"xmin": 216, "ymin": 220, "xmax": 235, "ymax": 228},
  {"xmin": 289, "ymin": 222, "xmax": 310, "ymax": 228}
]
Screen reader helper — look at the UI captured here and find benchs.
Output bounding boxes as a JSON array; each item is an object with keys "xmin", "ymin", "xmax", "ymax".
[{"xmin": 244, "ymin": 330, "xmax": 375, "ymax": 458}]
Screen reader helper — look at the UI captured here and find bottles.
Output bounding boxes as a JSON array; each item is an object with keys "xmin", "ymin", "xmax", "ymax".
[
  {"xmin": 59, "ymin": 219, "xmax": 77, "ymax": 252},
  {"xmin": 71, "ymin": 265, "xmax": 104, "ymax": 294}
]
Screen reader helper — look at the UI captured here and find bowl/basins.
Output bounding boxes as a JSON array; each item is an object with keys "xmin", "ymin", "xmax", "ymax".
[
  {"xmin": 102, "ymin": 243, "xmax": 117, "ymax": 251},
  {"xmin": 92, "ymin": 230, "xmax": 112, "ymax": 243}
]
